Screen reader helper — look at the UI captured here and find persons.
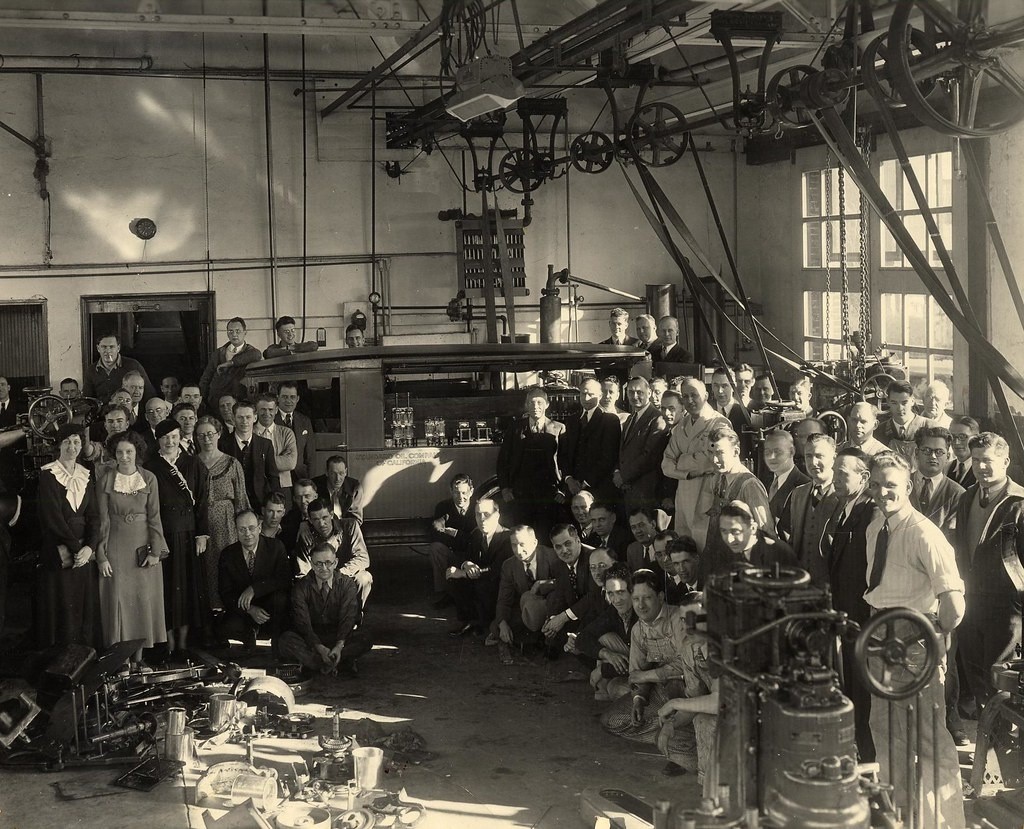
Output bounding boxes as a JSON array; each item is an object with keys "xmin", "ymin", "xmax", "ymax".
[
  {"xmin": 428, "ymin": 363, "xmax": 1024, "ymax": 798},
  {"xmin": 80, "ymin": 330, "xmax": 156, "ymax": 402},
  {"xmin": 0, "ymin": 377, "xmax": 375, "ymax": 674},
  {"xmin": 631, "ymin": 314, "xmax": 658, "ymax": 349},
  {"xmin": 263, "ymin": 316, "xmax": 319, "ymax": 360},
  {"xmin": 861, "ymin": 450, "xmax": 966, "ymax": 829},
  {"xmin": 199, "ymin": 317, "xmax": 261, "ymax": 408},
  {"xmin": 598, "ymin": 308, "xmax": 642, "ymax": 348},
  {"xmin": 345, "ymin": 324, "xmax": 365, "ymax": 348},
  {"xmin": 647, "ymin": 316, "xmax": 696, "ymax": 376}
]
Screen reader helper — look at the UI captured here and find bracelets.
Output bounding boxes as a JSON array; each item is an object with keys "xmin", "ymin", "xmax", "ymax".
[
  {"xmin": 230, "ymin": 359, "xmax": 234, "ymax": 366},
  {"xmin": 286, "ymin": 344, "xmax": 289, "ymax": 351}
]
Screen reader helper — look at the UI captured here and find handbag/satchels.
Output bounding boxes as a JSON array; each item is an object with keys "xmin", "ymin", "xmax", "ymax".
[
  {"xmin": 56, "ymin": 537, "xmax": 96, "ymax": 569},
  {"xmin": 137, "ymin": 542, "xmax": 169, "ymax": 567}
]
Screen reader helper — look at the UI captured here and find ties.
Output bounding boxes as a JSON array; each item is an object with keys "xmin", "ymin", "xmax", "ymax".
[
  {"xmin": 570, "ymin": 565, "xmax": 576, "ymax": 591},
  {"xmin": 578, "ymin": 410, "xmax": 588, "ymax": 445},
  {"xmin": 623, "ymin": 412, "xmax": 638, "ymax": 444},
  {"xmin": 919, "ymin": 463, "xmax": 966, "ymax": 514},
  {"xmin": 242, "ymin": 440, "xmax": 248, "ymax": 452},
  {"xmin": 286, "ymin": 413, "xmax": 291, "ymax": 426},
  {"xmin": 187, "ymin": 439, "xmax": 194, "ymax": 455},
  {"xmin": 722, "ymin": 406, "xmax": 726, "ymax": 416},
  {"xmin": 769, "ymin": 475, "xmax": 889, "ymax": 594},
  {"xmin": 581, "ymin": 527, "xmax": 694, "ymax": 591},
  {"xmin": 321, "ymin": 581, "xmax": 328, "ymax": 606},
  {"xmin": 249, "ymin": 550, "xmax": 255, "ymax": 576},
  {"xmin": 524, "ymin": 552, "xmax": 534, "ymax": 581}
]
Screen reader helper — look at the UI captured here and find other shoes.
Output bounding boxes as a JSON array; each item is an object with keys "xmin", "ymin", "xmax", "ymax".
[
  {"xmin": 432, "ymin": 595, "xmax": 455, "ymax": 610},
  {"xmin": 165, "ymin": 648, "xmax": 190, "ymax": 664},
  {"xmin": 950, "ymin": 728, "xmax": 971, "ymax": 746},
  {"xmin": 663, "ymin": 761, "xmax": 687, "ymax": 775},
  {"xmin": 958, "ymin": 702, "xmax": 983, "ymax": 720},
  {"xmin": 131, "ymin": 658, "xmax": 152, "ymax": 674}
]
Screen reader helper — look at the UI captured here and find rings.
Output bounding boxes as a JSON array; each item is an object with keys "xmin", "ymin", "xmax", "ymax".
[{"xmin": 154, "ymin": 565, "xmax": 157, "ymax": 567}]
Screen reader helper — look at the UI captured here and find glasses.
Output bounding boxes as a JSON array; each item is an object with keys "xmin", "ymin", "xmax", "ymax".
[
  {"xmin": 239, "ymin": 524, "xmax": 259, "ymax": 533},
  {"xmin": 312, "ymin": 558, "xmax": 335, "ymax": 568},
  {"xmin": 919, "ymin": 433, "xmax": 974, "ymax": 457}
]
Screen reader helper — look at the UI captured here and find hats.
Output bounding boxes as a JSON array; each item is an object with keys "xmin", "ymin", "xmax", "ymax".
[{"xmin": 155, "ymin": 418, "xmax": 180, "ymax": 437}]
